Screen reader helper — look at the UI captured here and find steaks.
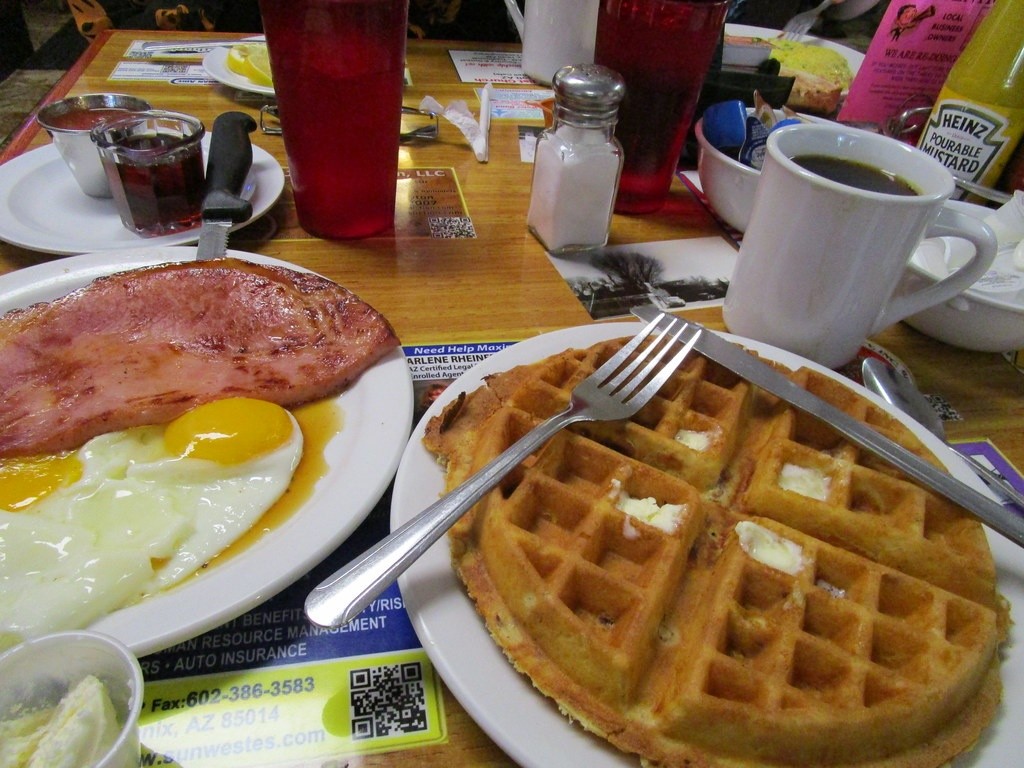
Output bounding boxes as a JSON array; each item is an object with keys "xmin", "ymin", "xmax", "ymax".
[{"xmin": 0, "ymin": 256, "xmax": 401, "ymax": 457}]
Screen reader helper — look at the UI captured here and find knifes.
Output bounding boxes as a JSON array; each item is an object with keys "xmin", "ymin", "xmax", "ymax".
[
  {"xmin": 631, "ymin": 305, "xmax": 1024, "ymax": 547},
  {"xmin": 198, "ymin": 111, "xmax": 256, "ymax": 260}
]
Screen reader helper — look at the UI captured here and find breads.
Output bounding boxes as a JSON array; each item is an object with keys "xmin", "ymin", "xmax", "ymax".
[
  {"xmin": 775, "ymin": 68, "xmax": 842, "ymax": 115},
  {"xmin": 769, "ymin": 41, "xmax": 854, "ymax": 92},
  {"xmin": 422, "ymin": 337, "xmax": 1012, "ymax": 768}
]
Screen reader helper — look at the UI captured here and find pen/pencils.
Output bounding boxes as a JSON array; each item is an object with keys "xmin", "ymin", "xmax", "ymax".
[
  {"xmin": 473, "ymin": 87, "xmax": 492, "ymax": 163},
  {"xmin": 151, "ymin": 53, "xmax": 205, "ymax": 62}
]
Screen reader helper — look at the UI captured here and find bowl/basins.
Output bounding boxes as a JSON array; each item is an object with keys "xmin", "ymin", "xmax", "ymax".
[
  {"xmin": 695, "ymin": 108, "xmax": 844, "ymax": 235},
  {"xmin": 0, "ymin": 629, "xmax": 146, "ymax": 768},
  {"xmin": 893, "ymin": 200, "xmax": 1024, "ymax": 355},
  {"xmin": 35, "ymin": 92, "xmax": 154, "ymax": 199}
]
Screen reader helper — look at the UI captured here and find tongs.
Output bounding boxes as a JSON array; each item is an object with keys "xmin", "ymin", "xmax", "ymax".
[{"xmin": 261, "ymin": 103, "xmax": 441, "ymax": 136}]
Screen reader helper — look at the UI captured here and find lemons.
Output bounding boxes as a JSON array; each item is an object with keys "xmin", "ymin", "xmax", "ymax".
[{"xmin": 228, "ymin": 43, "xmax": 273, "ymax": 88}]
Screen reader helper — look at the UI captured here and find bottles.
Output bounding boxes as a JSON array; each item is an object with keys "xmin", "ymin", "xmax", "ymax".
[
  {"xmin": 525, "ymin": 61, "xmax": 625, "ymax": 254},
  {"xmin": 916, "ymin": 0, "xmax": 1024, "ymax": 206}
]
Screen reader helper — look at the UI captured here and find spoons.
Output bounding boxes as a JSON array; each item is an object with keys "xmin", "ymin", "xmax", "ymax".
[{"xmin": 861, "ymin": 357, "xmax": 1024, "ymax": 510}]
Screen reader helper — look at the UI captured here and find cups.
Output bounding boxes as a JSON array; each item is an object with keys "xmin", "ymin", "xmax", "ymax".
[
  {"xmin": 90, "ymin": 108, "xmax": 206, "ymax": 238},
  {"xmin": 506, "ymin": 0, "xmax": 599, "ymax": 87},
  {"xmin": 722, "ymin": 123, "xmax": 999, "ymax": 365},
  {"xmin": 594, "ymin": 1, "xmax": 727, "ymax": 213},
  {"xmin": 258, "ymin": 1, "xmax": 407, "ymax": 241}
]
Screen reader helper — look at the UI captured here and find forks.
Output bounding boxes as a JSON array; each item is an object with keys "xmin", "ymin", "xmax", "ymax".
[
  {"xmin": 779, "ymin": 0, "xmax": 835, "ymax": 43},
  {"xmin": 303, "ymin": 313, "xmax": 704, "ymax": 628}
]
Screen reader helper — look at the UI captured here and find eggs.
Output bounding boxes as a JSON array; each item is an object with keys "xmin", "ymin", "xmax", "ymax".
[
  {"xmin": 0, "ymin": 445, "xmax": 159, "ymax": 652},
  {"xmin": 81, "ymin": 397, "xmax": 304, "ymax": 592}
]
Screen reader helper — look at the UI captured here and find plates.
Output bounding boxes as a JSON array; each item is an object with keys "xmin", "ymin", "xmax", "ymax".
[
  {"xmin": 724, "ymin": 23, "xmax": 866, "ymax": 72},
  {"xmin": 386, "ymin": 323, "xmax": 1024, "ymax": 768},
  {"xmin": 0, "ymin": 249, "xmax": 415, "ymax": 669},
  {"xmin": 201, "ymin": 34, "xmax": 276, "ymax": 97},
  {"xmin": 0, "ymin": 131, "xmax": 285, "ymax": 257}
]
